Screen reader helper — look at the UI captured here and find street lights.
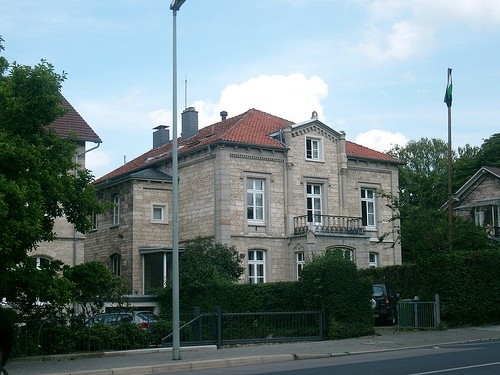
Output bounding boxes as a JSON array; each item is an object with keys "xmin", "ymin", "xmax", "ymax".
[{"xmin": 170, "ymin": 0, "xmax": 185, "ymax": 360}]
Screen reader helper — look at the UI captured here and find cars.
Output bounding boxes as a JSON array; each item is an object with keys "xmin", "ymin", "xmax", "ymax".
[{"xmin": 86, "ymin": 310, "xmax": 162, "ymax": 334}]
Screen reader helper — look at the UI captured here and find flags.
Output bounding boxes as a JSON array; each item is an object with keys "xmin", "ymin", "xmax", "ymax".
[{"xmin": 444, "ymin": 67, "xmax": 454, "ymax": 107}]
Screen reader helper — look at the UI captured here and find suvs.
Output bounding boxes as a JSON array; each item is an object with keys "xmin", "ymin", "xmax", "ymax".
[{"xmin": 371, "ymin": 285, "xmax": 402, "ymax": 326}]
